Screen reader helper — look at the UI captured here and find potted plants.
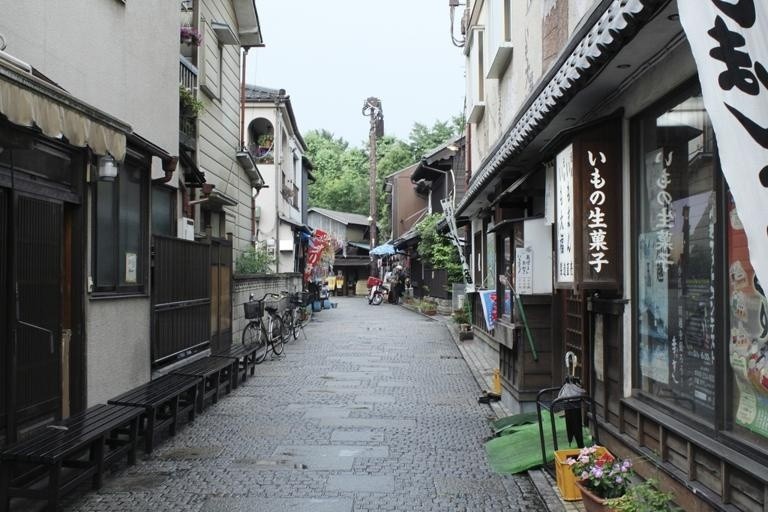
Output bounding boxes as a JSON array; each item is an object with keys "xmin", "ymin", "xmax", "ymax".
[{"xmin": 420, "ymin": 302, "xmax": 436, "ymax": 315}]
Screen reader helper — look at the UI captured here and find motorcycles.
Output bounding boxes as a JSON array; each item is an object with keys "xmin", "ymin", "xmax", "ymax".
[{"xmin": 366, "ymin": 277, "xmax": 384, "ymax": 305}]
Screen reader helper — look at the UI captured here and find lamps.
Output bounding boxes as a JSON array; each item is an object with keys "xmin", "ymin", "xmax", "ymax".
[{"xmin": 99, "ymin": 154, "xmax": 119, "ymax": 182}]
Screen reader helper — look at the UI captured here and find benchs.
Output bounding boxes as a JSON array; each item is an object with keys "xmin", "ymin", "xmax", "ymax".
[
  {"xmin": 165, "ymin": 355, "xmax": 237, "ymax": 415},
  {"xmin": 104, "ymin": 372, "xmax": 206, "ymax": 456},
  {"xmin": 0, "ymin": 402, "xmax": 149, "ymax": 512},
  {"xmin": 209, "ymin": 346, "xmax": 260, "ymax": 389}
]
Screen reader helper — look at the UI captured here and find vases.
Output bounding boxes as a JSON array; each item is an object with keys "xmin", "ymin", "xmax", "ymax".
[
  {"xmin": 571, "ymin": 445, "xmax": 633, "ymax": 497},
  {"xmin": 574, "ymin": 480, "xmax": 633, "ymax": 512}
]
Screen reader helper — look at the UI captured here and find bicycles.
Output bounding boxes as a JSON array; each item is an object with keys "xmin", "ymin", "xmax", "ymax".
[
  {"xmin": 280, "ymin": 290, "xmax": 312, "ymax": 345},
  {"xmin": 241, "ymin": 293, "xmax": 286, "ymax": 365}
]
